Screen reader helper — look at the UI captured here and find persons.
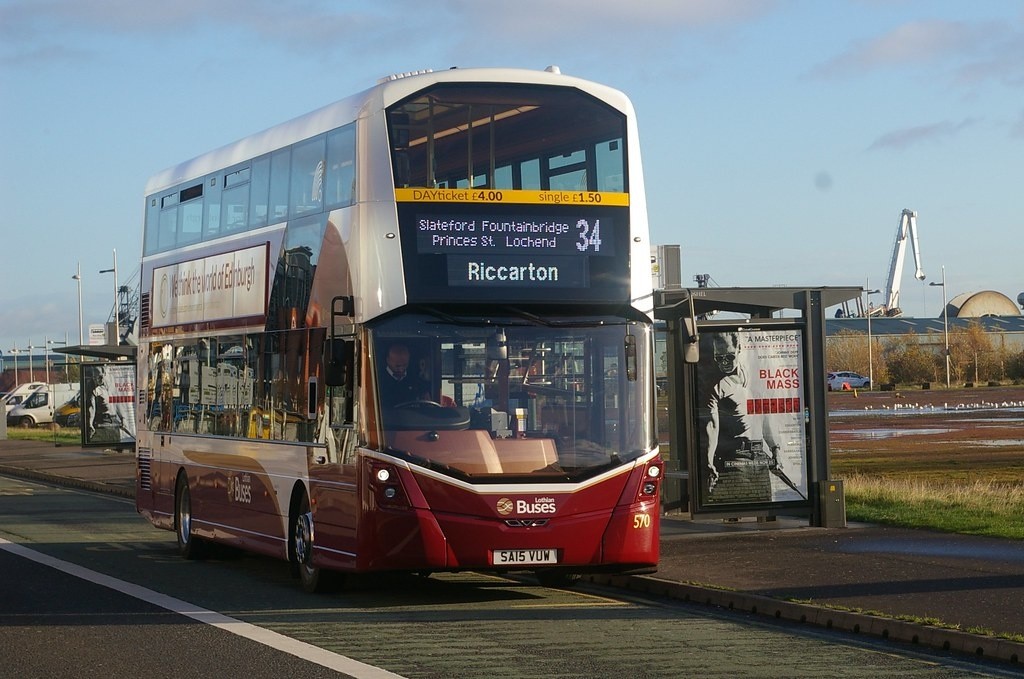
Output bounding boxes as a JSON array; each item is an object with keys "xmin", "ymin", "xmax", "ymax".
[
  {"xmin": 380, "ymin": 344, "xmax": 430, "ymax": 412},
  {"xmin": 87, "ymin": 367, "xmax": 127, "ymax": 442},
  {"xmin": 702, "ymin": 334, "xmax": 784, "ymax": 503}
]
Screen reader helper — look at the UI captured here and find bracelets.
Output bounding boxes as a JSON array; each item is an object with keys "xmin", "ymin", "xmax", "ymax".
[
  {"xmin": 120, "ymin": 416, "xmax": 126, "ymax": 420},
  {"xmin": 770, "ymin": 444, "xmax": 780, "ymax": 452}
]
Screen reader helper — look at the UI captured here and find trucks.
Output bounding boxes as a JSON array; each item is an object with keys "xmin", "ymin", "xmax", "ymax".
[
  {"xmin": 7, "ymin": 382, "xmax": 80, "ymax": 429},
  {"xmin": 1, "ymin": 382, "xmax": 46, "ymax": 415}
]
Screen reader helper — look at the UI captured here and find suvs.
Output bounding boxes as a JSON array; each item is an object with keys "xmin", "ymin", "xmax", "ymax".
[{"xmin": 827, "ymin": 371, "xmax": 873, "ymax": 391}]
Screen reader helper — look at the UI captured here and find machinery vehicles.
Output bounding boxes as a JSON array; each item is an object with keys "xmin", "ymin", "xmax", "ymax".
[{"xmin": 868, "ymin": 208, "xmax": 926, "ymax": 317}]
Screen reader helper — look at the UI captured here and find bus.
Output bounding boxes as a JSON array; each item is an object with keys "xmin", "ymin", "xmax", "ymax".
[{"xmin": 137, "ymin": 69, "xmax": 701, "ymax": 595}]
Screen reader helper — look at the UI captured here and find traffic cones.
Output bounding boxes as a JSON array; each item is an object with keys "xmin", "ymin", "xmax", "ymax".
[
  {"xmin": 854, "ymin": 388, "xmax": 859, "ymax": 400},
  {"xmin": 895, "ymin": 392, "xmax": 905, "ymax": 399}
]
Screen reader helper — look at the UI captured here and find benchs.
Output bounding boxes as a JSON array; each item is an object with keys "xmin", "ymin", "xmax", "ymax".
[{"xmin": 662, "ymin": 470, "xmax": 740, "ymax": 523}]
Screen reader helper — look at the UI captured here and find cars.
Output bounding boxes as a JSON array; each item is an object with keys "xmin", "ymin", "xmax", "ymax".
[{"xmin": 53, "ymin": 391, "xmax": 81, "ymax": 428}]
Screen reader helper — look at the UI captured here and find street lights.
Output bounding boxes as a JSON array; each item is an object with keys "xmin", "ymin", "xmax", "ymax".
[
  {"xmin": 72, "ymin": 262, "xmax": 83, "ymax": 362},
  {"xmin": 929, "ymin": 282, "xmax": 951, "ymax": 386},
  {"xmin": 8, "ymin": 342, "xmax": 17, "ymax": 387},
  {"xmin": 28, "ymin": 336, "xmax": 49, "ymax": 384},
  {"xmin": 866, "ymin": 277, "xmax": 883, "ymax": 390},
  {"xmin": 12, "ymin": 339, "xmax": 32, "ymax": 382},
  {"xmin": 48, "ymin": 332, "xmax": 69, "ymax": 383},
  {"xmin": 99, "ymin": 249, "xmax": 119, "ymax": 345}
]
